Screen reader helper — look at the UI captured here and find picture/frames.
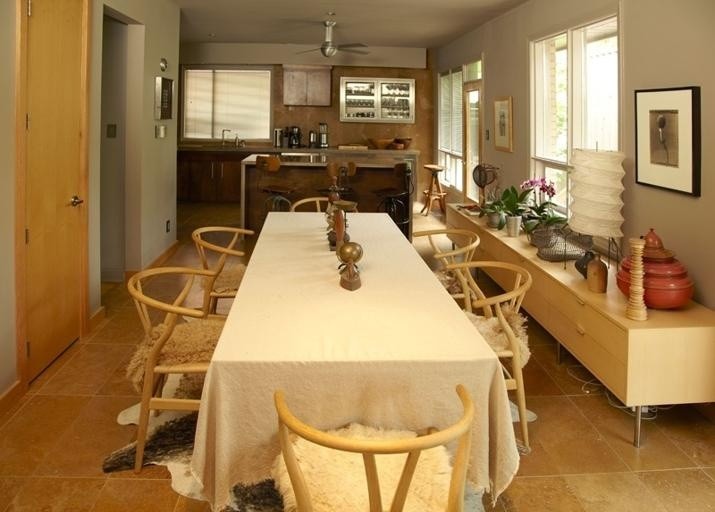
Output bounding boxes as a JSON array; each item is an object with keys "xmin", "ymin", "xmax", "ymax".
[
  {"xmin": 634, "ymin": 87, "xmax": 702, "ymax": 198},
  {"xmin": 492, "ymin": 96, "xmax": 512, "ymax": 153}
]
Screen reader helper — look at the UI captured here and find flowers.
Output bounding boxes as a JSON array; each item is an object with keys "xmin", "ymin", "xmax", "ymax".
[{"xmin": 521, "ymin": 177, "xmax": 568, "ymax": 234}]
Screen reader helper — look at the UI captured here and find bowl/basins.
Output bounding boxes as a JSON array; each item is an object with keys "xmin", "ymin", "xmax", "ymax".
[
  {"xmin": 395, "ymin": 137, "xmax": 411, "ymax": 150},
  {"xmin": 369, "ymin": 137, "xmax": 394, "ymax": 149},
  {"xmin": 392, "ymin": 143, "xmax": 404, "ymax": 149}
]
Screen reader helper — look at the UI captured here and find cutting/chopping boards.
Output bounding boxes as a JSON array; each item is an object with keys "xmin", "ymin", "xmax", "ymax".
[{"xmin": 338, "ymin": 145, "xmax": 368, "ymax": 151}]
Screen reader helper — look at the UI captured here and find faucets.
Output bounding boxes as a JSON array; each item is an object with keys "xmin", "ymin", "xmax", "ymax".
[{"xmin": 221, "ymin": 129, "xmax": 231, "ymax": 146}]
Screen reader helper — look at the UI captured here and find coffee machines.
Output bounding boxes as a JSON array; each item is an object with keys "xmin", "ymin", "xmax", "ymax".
[{"xmin": 319, "ymin": 122, "xmax": 329, "ymax": 147}]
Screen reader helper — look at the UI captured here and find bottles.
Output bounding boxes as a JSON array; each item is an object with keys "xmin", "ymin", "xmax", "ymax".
[
  {"xmin": 385, "ymin": 108, "xmax": 409, "ymax": 118},
  {"xmin": 273, "ymin": 128, "xmax": 283, "ymax": 148},
  {"xmin": 585, "ymin": 252, "xmax": 610, "ymax": 294},
  {"xmin": 386, "ymin": 83, "xmax": 409, "ymax": 97}
]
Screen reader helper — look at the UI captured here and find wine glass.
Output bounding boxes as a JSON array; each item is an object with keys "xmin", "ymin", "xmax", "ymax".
[{"xmin": 345, "ymin": 99, "xmax": 374, "ymax": 106}]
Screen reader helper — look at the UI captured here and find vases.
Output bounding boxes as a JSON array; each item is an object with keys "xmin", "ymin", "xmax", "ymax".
[{"xmin": 530, "ymin": 225, "xmax": 555, "ymax": 248}]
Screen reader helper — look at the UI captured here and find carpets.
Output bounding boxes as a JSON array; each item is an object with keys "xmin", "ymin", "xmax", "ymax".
[{"xmin": 103, "ymin": 315, "xmax": 537, "ymax": 512}]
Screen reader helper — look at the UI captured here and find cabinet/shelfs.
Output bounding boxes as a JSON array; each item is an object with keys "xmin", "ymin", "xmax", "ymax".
[
  {"xmin": 282, "ymin": 65, "xmax": 333, "ymax": 106},
  {"xmin": 339, "ymin": 76, "xmax": 416, "ymax": 124}
]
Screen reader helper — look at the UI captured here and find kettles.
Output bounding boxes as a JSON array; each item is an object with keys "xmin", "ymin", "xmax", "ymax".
[{"xmin": 289, "ymin": 126, "xmax": 302, "ymax": 148}]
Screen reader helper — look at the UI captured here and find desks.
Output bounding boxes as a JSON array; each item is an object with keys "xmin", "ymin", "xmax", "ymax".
[{"xmin": 446, "ymin": 203, "xmax": 715, "ymax": 447}]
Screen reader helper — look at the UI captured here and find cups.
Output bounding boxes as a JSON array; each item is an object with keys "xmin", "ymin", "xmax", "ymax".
[
  {"xmin": 345, "ymin": 111, "xmax": 373, "ymax": 118},
  {"xmin": 346, "ymin": 86, "xmax": 370, "ymax": 94}
]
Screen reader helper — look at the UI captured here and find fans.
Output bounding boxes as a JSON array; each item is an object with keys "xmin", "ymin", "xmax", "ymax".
[{"xmin": 294, "ymin": 11, "xmax": 371, "ymax": 58}]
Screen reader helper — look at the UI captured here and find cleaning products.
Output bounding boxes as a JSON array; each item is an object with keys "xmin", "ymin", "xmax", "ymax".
[{"xmin": 235, "ymin": 134, "xmax": 239, "ymax": 147}]
